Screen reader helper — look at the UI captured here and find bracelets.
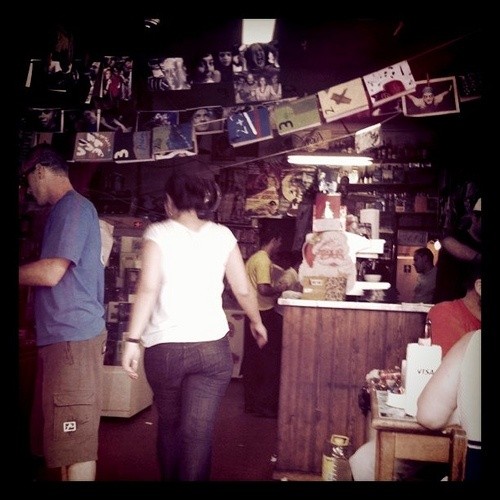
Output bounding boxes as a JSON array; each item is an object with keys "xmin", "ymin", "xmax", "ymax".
[{"xmin": 126, "ymin": 337, "xmax": 141, "ymax": 344}]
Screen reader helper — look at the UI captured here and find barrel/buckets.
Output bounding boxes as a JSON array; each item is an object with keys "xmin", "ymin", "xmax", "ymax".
[{"xmin": 322, "ymin": 434, "xmax": 355, "ymax": 481}]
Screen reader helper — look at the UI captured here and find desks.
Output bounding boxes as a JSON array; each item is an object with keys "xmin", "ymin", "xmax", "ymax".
[{"xmin": 367, "ymin": 368, "xmax": 468, "ymax": 481}]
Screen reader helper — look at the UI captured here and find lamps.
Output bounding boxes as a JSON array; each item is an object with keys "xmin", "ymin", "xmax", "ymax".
[{"xmin": 284, "ymin": 150, "xmax": 376, "ymax": 168}]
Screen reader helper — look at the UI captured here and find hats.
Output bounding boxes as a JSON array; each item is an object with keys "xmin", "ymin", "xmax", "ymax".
[{"xmin": 17, "ymin": 139, "xmax": 65, "ymax": 177}]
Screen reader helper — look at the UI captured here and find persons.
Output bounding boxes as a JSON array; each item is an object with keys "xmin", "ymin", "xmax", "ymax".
[
  {"xmin": 19, "ymin": 141, "xmax": 108, "ymax": 482},
  {"xmin": 434, "ymin": 199, "xmax": 483, "ymax": 306},
  {"xmin": 414, "ymin": 327, "xmax": 482, "ymax": 485},
  {"xmin": 21, "ymin": 41, "xmax": 457, "ymax": 216},
  {"xmin": 120, "ymin": 167, "xmax": 269, "ymax": 483},
  {"xmin": 243, "ymin": 220, "xmax": 304, "ymax": 419},
  {"xmin": 411, "ymin": 247, "xmax": 438, "ymax": 305},
  {"xmin": 426, "ymin": 256, "xmax": 483, "ymax": 363}
]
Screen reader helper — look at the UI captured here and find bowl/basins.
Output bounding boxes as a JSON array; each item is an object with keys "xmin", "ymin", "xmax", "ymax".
[{"xmin": 363, "ymin": 274, "xmax": 382, "ymax": 282}]
